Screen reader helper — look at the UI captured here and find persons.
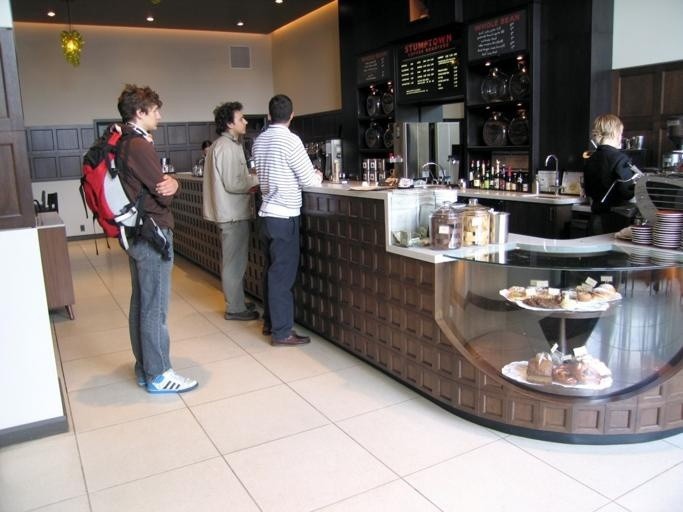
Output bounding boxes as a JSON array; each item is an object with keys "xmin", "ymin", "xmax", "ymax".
[
  {"xmin": 584, "ymin": 113, "xmax": 642, "ymax": 237},
  {"xmin": 201, "ymin": 101, "xmax": 261, "ymax": 322},
  {"xmin": 116, "ymin": 81, "xmax": 198, "ymax": 394},
  {"xmin": 250, "ymin": 94, "xmax": 323, "ymax": 347},
  {"xmin": 197, "ymin": 139, "xmax": 213, "ymax": 172}
]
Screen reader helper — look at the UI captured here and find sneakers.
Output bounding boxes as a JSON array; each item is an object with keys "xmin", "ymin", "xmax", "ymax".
[
  {"xmin": 224, "ymin": 303, "xmax": 259, "ymax": 320},
  {"xmin": 263, "ymin": 325, "xmax": 310, "ymax": 345},
  {"xmin": 137, "ymin": 368, "xmax": 198, "ymax": 393}
]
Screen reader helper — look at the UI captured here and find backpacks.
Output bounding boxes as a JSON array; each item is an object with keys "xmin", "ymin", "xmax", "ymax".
[{"xmin": 80, "ymin": 123, "xmax": 147, "ymax": 237}]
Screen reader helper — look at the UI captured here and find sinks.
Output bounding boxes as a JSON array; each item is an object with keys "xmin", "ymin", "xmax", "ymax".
[{"xmin": 523, "ymin": 195, "xmax": 568, "ymax": 200}]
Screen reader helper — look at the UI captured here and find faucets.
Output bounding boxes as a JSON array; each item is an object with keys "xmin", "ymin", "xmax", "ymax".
[
  {"xmin": 422, "ymin": 162, "xmax": 445, "ymax": 185},
  {"xmin": 545, "ymin": 154, "xmax": 560, "ymax": 196}
]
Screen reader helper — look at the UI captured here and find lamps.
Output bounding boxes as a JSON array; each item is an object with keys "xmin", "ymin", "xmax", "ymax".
[{"xmin": 58, "ymin": 0, "xmax": 85, "ymax": 68}]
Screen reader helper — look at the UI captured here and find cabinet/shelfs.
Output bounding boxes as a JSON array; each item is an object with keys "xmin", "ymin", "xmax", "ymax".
[
  {"xmin": 469, "ymin": 59, "xmax": 534, "ymax": 147},
  {"xmin": 357, "ymin": 86, "xmax": 395, "ymax": 178}
]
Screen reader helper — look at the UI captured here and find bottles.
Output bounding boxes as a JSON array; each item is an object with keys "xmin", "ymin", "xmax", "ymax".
[{"xmin": 469, "ymin": 159, "xmax": 530, "ymax": 192}]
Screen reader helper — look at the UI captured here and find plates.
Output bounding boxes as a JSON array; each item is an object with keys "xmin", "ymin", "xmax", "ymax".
[
  {"xmin": 626, "ymin": 251, "xmax": 683, "ymax": 268},
  {"xmin": 630, "ymin": 209, "xmax": 683, "ymax": 249}
]
